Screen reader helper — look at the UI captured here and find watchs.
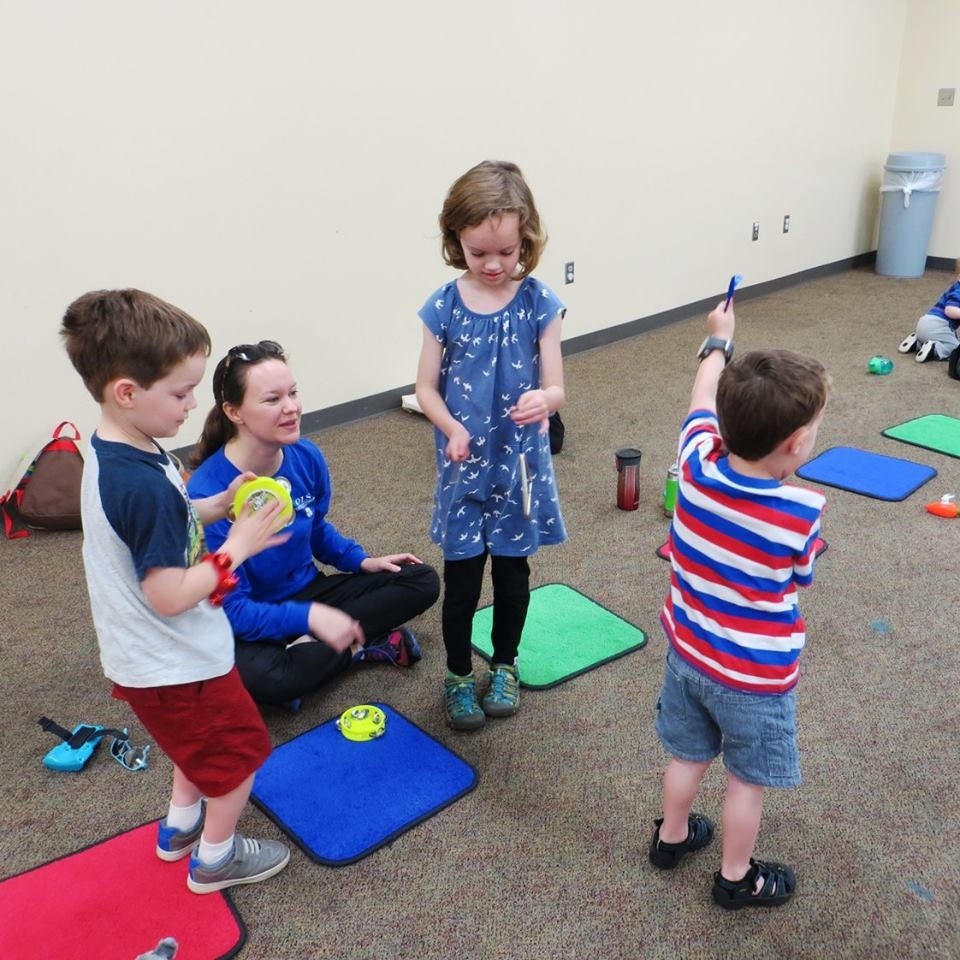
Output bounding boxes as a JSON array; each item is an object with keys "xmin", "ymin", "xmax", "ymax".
[{"xmin": 696, "ymin": 336, "xmax": 734, "ymax": 364}]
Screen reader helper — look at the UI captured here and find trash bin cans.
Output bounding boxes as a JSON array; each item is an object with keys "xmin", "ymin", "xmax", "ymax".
[{"xmin": 875, "ymin": 151, "xmax": 946, "ymax": 278}]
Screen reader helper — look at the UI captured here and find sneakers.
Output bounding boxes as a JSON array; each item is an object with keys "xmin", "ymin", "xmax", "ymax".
[
  {"xmin": 483, "ymin": 661, "xmax": 522, "ymax": 715},
  {"xmin": 443, "ymin": 669, "xmax": 484, "ymax": 731},
  {"xmin": 346, "ymin": 629, "xmax": 421, "ymax": 676},
  {"xmin": 187, "ymin": 834, "xmax": 291, "ymax": 894},
  {"xmin": 155, "ymin": 797, "xmax": 210, "ymax": 861}
]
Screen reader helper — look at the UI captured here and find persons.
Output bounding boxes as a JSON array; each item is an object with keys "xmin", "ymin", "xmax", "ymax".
[
  {"xmin": 899, "ymin": 275, "xmax": 960, "ymax": 363},
  {"xmin": 184, "ymin": 340, "xmax": 442, "ymax": 718},
  {"xmin": 63, "ymin": 289, "xmax": 291, "ymax": 894},
  {"xmin": 649, "ymin": 297, "xmax": 833, "ymax": 912},
  {"xmin": 415, "ymin": 160, "xmax": 564, "ymax": 731}
]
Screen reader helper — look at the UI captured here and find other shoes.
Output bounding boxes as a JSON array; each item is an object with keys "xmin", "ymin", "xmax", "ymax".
[
  {"xmin": 899, "ymin": 331, "xmax": 917, "ymax": 353},
  {"xmin": 915, "ymin": 339, "xmax": 932, "ymax": 362}
]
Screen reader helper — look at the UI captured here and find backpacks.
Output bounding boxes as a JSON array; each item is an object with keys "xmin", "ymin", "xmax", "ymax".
[{"xmin": 0, "ymin": 422, "xmax": 84, "ymax": 537}]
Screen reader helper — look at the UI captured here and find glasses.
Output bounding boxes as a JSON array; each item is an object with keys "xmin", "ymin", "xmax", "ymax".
[
  {"xmin": 221, "ymin": 340, "xmax": 282, "ymax": 403},
  {"xmin": 111, "ymin": 730, "xmax": 150, "ymax": 773}
]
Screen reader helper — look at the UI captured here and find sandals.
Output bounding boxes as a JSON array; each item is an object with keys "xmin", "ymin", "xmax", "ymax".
[
  {"xmin": 712, "ymin": 853, "xmax": 796, "ymax": 910},
  {"xmin": 649, "ymin": 813, "xmax": 714, "ymax": 869}
]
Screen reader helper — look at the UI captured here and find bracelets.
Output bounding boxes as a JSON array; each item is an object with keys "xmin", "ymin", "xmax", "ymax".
[{"xmin": 200, "ymin": 552, "xmax": 239, "ymax": 607}]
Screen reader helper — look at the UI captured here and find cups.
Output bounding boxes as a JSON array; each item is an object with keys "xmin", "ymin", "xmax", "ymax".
[
  {"xmin": 615, "ymin": 449, "xmax": 643, "ymax": 512},
  {"xmin": 664, "ymin": 463, "xmax": 680, "ymax": 518}
]
